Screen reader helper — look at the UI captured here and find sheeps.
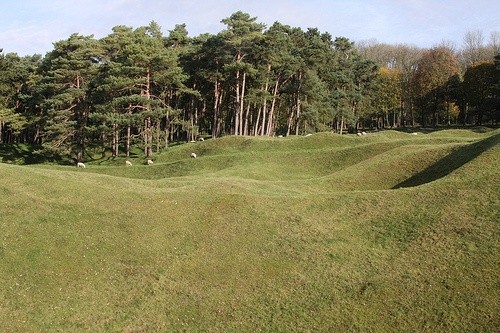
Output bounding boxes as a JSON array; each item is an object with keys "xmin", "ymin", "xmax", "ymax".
[
  {"xmin": 199, "ymin": 137, "xmax": 204, "ymax": 141},
  {"xmin": 144, "ymin": 159, "xmax": 154, "ymax": 165},
  {"xmin": 190, "ymin": 152, "xmax": 196, "ymax": 158},
  {"xmin": 362, "ymin": 131, "xmax": 366, "ymax": 135},
  {"xmin": 125, "ymin": 160, "xmax": 132, "ymax": 166},
  {"xmin": 357, "ymin": 132, "xmax": 361, "ymax": 136},
  {"xmin": 278, "ymin": 134, "xmax": 283, "ymax": 137},
  {"xmin": 77, "ymin": 162, "xmax": 85, "ymax": 168}
]
